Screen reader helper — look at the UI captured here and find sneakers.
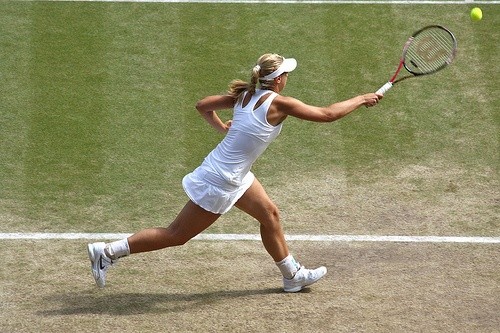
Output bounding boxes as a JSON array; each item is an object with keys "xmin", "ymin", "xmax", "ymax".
[
  {"xmin": 88, "ymin": 242, "xmax": 118, "ymax": 288},
  {"xmin": 283, "ymin": 266, "xmax": 327, "ymax": 292}
]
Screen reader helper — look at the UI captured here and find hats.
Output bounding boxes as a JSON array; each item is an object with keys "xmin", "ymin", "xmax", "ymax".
[{"xmin": 258, "ymin": 55, "xmax": 297, "ymax": 81}]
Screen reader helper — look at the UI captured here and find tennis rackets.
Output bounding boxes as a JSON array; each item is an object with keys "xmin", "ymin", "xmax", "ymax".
[{"xmin": 364, "ymin": 25, "xmax": 458, "ymax": 109}]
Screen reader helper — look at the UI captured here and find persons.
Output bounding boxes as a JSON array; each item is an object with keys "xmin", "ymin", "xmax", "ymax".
[{"xmin": 87, "ymin": 52, "xmax": 383, "ymax": 291}]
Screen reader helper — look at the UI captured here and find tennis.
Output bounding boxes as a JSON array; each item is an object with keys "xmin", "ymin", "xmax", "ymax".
[{"xmin": 470, "ymin": 7, "xmax": 483, "ymax": 21}]
